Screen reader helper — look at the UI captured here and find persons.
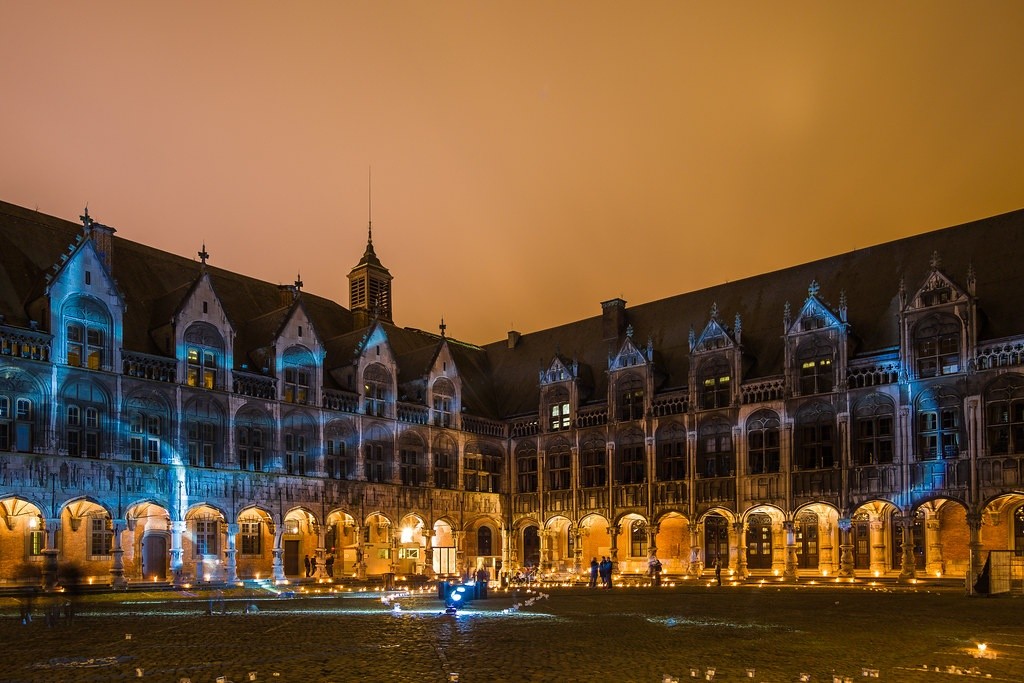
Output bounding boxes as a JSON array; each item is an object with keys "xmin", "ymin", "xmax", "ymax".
[
  {"xmin": 588, "ymin": 556, "xmax": 612, "ymax": 589},
  {"xmin": 304, "ymin": 555, "xmax": 311, "ymax": 578},
  {"xmin": 310, "ymin": 556, "xmax": 318, "ymax": 576},
  {"xmin": 325, "ymin": 554, "xmax": 334, "ymax": 577},
  {"xmin": 511, "ymin": 565, "xmax": 538, "ymax": 584},
  {"xmin": 712, "ymin": 556, "xmax": 722, "ymax": 586},
  {"xmin": 473, "ymin": 569, "xmax": 490, "ymax": 587}
]
